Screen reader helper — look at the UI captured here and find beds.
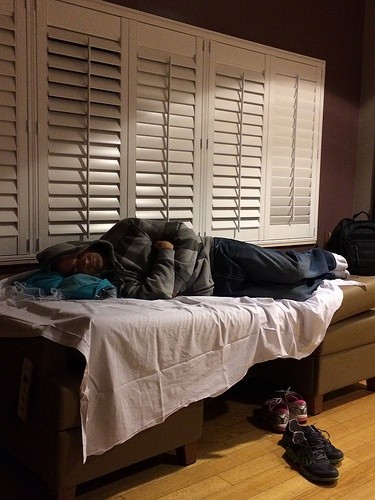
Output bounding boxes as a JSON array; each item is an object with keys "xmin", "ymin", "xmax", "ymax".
[{"xmin": 0, "ymin": 274, "xmax": 375, "ymax": 499}]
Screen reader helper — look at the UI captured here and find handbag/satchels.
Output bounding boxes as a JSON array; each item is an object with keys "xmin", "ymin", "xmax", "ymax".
[{"xmin": 325, "ymin": 210, "xmax": 374, "ymax": 276}]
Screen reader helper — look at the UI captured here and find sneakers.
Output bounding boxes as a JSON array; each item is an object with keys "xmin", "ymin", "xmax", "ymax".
[
  {"xmin": 276, "ymin": 388, "xmax": 309, "ymax": 422},
  {"xmin": 284, "ymin": 431, "xmax": 340, "ymax": 481},
  {"xmin": 263, "ymin": 396, "xmax": 290, "ymax": 431},
  {"xmin": 281, "ymin": 419, "xmax": 344, "ymax": 462}
]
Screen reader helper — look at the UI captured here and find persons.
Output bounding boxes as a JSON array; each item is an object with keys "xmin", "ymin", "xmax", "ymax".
[{"xmin": 35, "ymin": 218, "xmax": 350, "ymax": 303}]
